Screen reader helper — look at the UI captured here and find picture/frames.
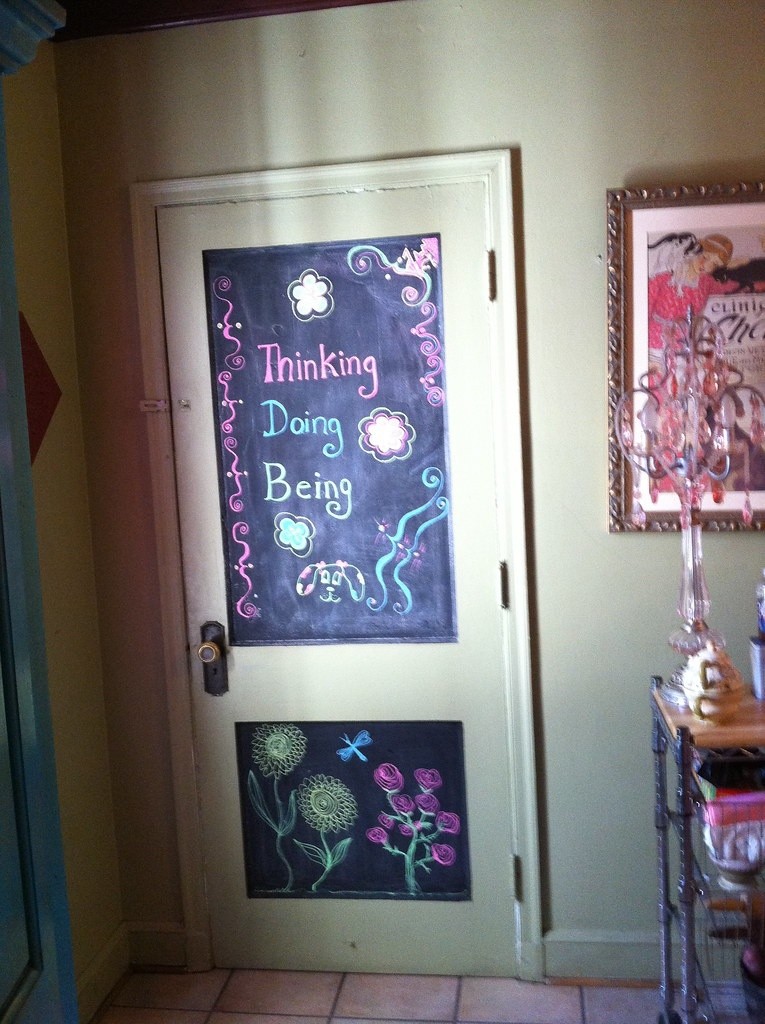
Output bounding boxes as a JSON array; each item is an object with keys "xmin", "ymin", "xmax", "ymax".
[{"xmin": 605, "ymin": 180, "xmax": 765, "ymax": 536}]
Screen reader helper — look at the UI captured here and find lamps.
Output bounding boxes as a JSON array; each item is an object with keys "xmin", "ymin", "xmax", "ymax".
[{"xmin": 612, "ymin": 302, "xmax": 765, "ymax": 704}]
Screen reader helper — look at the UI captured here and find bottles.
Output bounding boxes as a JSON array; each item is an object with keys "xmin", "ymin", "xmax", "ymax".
[{"xmin": 756, "ymin": 568, "xmax": 765, "ymax": 641}]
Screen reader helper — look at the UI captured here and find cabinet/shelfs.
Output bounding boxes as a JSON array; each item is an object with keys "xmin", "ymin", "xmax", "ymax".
[{"xmin": 646, "ymin": 673, "xmax": 765, "ymax": 1024}]
